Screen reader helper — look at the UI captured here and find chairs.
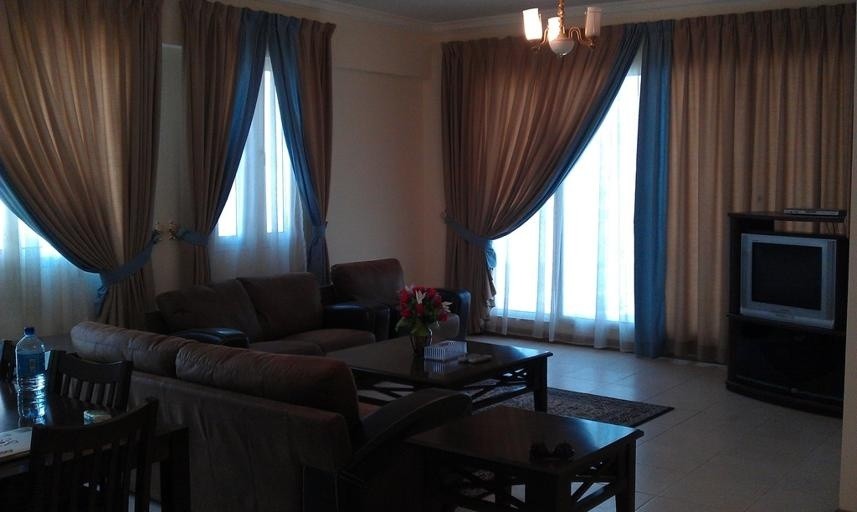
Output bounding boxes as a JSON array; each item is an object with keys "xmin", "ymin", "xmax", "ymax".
[
  {"xmin": 0, "ymin": 335, "xmax": 192, "ymax": 511},
  {"xmin": 319, "ymin": 257, "xmax": 473, "ymax": 337}
]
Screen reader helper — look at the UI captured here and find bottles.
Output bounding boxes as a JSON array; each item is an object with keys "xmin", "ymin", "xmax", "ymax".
[{"xmin": 12, "ymin": 325, "xmax": 48, "ymax": 419}]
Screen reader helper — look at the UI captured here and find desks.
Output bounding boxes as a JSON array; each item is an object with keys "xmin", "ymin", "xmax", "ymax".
[{"xmin": 399, "ymin": 402, "xmax": 645, "ymax": 511}]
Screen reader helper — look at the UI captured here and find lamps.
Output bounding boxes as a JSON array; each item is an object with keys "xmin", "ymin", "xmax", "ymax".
[{"xmin": 517, "ymin": 1, "xmax": 604, "ymax": 60}]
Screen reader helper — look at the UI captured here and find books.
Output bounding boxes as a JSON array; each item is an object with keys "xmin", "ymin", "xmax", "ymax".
[{"xmin": 0, "ymin": 427, "xmax": 33, "ymax": 460}]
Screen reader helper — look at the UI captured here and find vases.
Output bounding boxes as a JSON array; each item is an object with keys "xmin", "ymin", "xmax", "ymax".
[{"xmin": 408, "ymin": 326, "xmax": 433, "ymax": 356}]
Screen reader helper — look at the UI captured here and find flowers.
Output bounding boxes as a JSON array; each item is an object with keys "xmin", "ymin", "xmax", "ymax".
[{"xmin": 396, "ymin": 283, "xmax": 454, "ymax": 338}]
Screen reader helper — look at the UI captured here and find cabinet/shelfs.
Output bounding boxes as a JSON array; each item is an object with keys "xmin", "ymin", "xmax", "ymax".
[{"xmin": 725, "ymin": 208, "xmax": 849, "ymax": 420}]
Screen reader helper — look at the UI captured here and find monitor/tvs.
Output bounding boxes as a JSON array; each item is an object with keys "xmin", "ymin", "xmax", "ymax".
[{"xmin": 740, "ymin": 232, "xmax": 846, "ymax": 331}]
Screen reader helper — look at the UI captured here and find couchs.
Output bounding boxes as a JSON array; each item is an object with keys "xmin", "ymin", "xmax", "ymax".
[
  {"xmin": 63, "ymin": 322, "xmax": 468, "ymax": 512},
  {"xmin": 146, "ymin": 272, "xmax": 381, "ymax": 364}
]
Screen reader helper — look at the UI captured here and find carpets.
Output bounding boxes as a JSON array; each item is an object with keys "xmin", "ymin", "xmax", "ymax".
[{"xmin": 463, "ymin": 377, "xmax": 676, "ymax": 428}]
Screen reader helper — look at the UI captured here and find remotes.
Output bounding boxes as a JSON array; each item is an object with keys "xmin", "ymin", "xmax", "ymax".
[
  {"xmin": 458, "ymin": 353, "xmax": 480, "ymax": 363},
  {"xmin": 469, "ymin": 354, "xmax": 491, "ymax": 363}
]
